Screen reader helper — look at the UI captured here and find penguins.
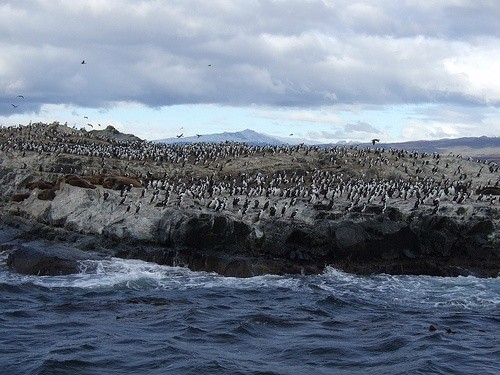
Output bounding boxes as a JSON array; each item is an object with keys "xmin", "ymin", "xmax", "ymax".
[{"xmin": 0, "ymin": 121, "xmax": 500, "ymax": 220}]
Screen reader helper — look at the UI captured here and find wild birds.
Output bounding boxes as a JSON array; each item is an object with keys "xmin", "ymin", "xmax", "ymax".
[
  {"xmin": 17, "ymin": 95, "xmax": 24, "ymax": 99},
  {"xmin": 81, "ymin": 60, "xmax": 87, "ymax": 64},
  {"xmin": 11, "ymin": 103, "xmax": 20, "ymax": 108},
  {"xmin": 83, "ymin": 116, "xmax": 88, "ymax": 119}
]
